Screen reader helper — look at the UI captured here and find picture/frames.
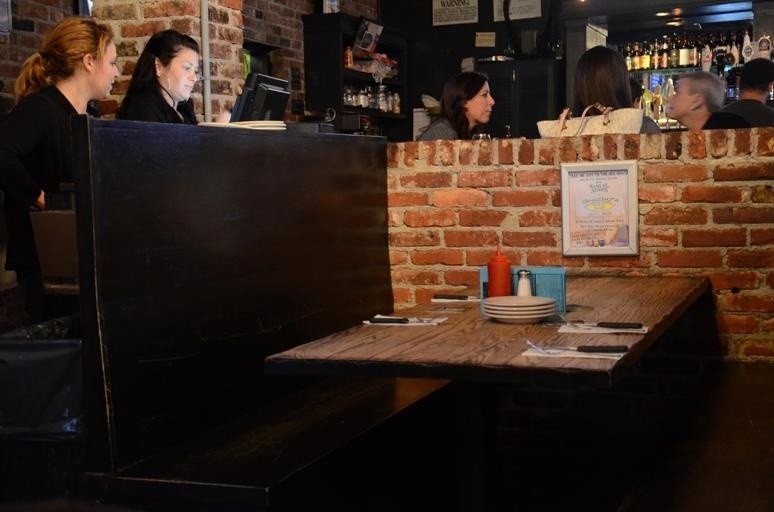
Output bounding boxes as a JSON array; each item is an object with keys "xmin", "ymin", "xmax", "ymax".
[{"xmin": 561, "ymin": 161, "xmax": 640, "ymax": 257}]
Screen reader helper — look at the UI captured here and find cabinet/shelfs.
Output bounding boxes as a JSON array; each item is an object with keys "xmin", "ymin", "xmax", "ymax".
[{"xmin": 304, "ymin": 11, "xmax": 412, "ymax": 118}]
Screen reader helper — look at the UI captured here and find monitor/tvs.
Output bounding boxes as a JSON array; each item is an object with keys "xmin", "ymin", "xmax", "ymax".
[{"xmin": 231, "ymin": 71, "xmax": 291, "ymax": 121}]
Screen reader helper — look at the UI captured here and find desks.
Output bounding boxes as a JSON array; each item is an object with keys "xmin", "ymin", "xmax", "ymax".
[{"xmin": 260, "ymin": 273, "xmax": 712, "ymax": 511}]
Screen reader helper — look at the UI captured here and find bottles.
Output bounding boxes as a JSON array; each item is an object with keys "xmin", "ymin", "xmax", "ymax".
[
  {"xmin": 726, "ymin": 70, "xmax": 737, "ymax": 103},
  {"xmin": 488, "ymin": 244, "xmax": 533, "ymax": 297},
  {"xmin": 343, "ymin": 85, "xmax": 401, "ymax": 114},
  {"xmin": 618, "ymin": 30, "xmax": 772, "ymax": 71}
]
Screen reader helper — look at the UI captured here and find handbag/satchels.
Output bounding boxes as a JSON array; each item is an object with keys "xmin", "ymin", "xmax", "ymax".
[{"xmin": 537, "ymin": 108, "xmax": 645, "ymax": 138}]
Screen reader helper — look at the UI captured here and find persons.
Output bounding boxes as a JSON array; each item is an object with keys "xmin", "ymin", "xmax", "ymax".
[
  {"xmin": 1, "ymin": 15, "xmax": 121, "ymax": 325},
  {"xmin": 415, "ymin": 72, "xmax": 495, "ymax": 139},
  {"xmin": 121, "ymin": 29, "xmax": 201, "ymax": 125},
  {"xmin": 669, "ymin": 69, "xmax": 751, "ymax": 128},
  {"xmin": 560, "ymin": 45, "xmax": 662, "ymax": 135},
  {"xmin": 723, "ymin": 58, "xmax": 774, "ymax": 126}
]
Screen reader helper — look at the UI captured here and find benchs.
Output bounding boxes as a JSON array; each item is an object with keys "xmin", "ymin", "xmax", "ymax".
[
  {"xmin": 654, "ymin": 358, "xmax": 774, "ymax": 509},
  {"xmin": 67, "ymin": 115, "xmax": 454, "ymax": 504}
]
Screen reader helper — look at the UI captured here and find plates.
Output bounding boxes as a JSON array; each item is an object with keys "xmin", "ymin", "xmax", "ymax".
[{"xmin": 482, "ymin": 295, "xmax": 558, "ymax": 326}]
